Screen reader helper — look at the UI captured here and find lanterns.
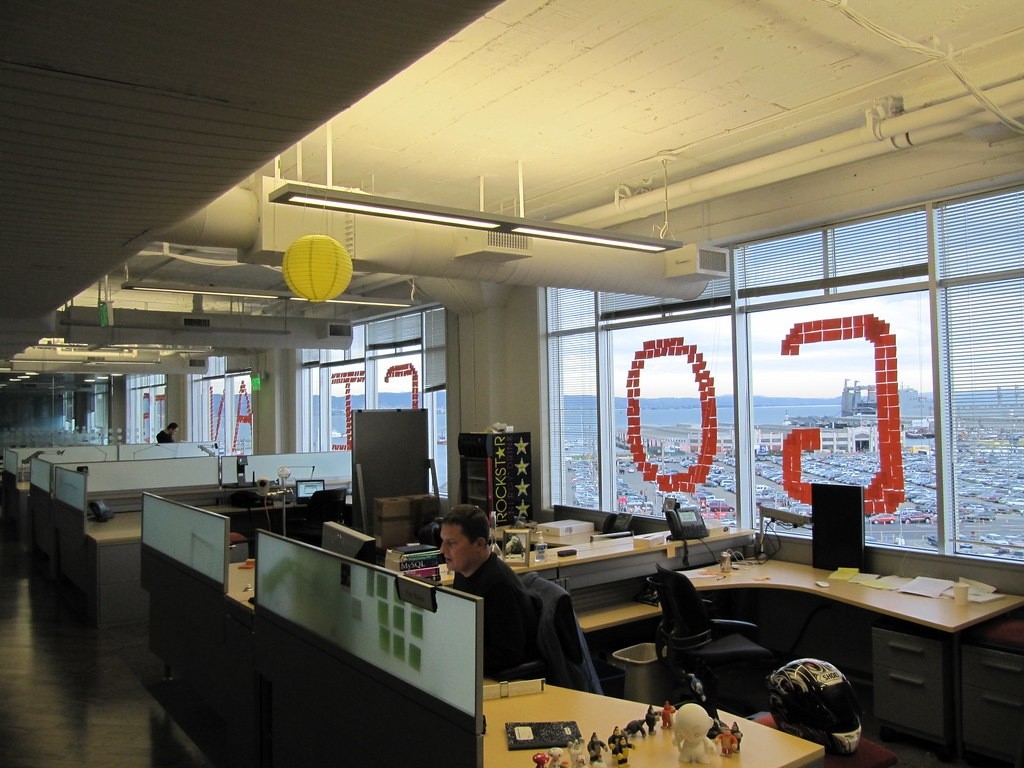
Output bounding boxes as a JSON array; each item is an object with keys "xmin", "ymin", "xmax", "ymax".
[{"xmin": 281, "ymin": 235, "xmax": 352, "ymax": 302}]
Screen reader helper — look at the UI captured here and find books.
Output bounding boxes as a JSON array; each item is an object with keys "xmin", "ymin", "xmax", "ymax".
[
  {"xmin": 506, "ymin": 721, "xmax": 581, "ymax": 751},
  {"xmin": 385, "ymin": 544, "xmax": 442, "ymax": 583}
]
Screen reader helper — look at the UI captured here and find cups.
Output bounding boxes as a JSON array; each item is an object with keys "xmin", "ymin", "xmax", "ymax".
[{"xmin": 953, "ymin": 583, "xmax": 970, "ymax": 606}]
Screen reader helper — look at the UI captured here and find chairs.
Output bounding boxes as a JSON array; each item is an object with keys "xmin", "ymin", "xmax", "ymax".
[
  {"xmin": 287, "ymin": 489, "xmax": 347, "ymax": 536},
  {"xmin": 497, "ymin": 571, "xmax": 605, "ymax": 696},
  {"xmin": 646, "ymin": 563, "xmax": 774, "ymax": 718}
]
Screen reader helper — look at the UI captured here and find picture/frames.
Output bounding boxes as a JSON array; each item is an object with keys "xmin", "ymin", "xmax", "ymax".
[{"xmin": 503, "ymin": 529, "xmax": 531, "ymax": 567}]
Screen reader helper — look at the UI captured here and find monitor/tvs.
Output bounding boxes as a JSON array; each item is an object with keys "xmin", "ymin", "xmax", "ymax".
[{"xmin": 321, "ymin": 522, "xmax": 377, "ymax": 566}]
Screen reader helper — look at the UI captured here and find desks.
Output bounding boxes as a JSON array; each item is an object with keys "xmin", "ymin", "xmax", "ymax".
[
  {"xmin": 16, "ymin": 480, "xmax": 31, "ymax": 543},
  {"xmin": 82, "ymin": 493, "xmax": 354, "ymax": 630},
  {"xmin": 401, "ymin": 523, "xmax": 1024, "ymax": 767}
]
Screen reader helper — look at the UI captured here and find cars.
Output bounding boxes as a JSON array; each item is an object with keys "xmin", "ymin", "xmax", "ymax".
[{"xmin": 565, "ymin": 417, "xmax": 1024, "ymax": 561}]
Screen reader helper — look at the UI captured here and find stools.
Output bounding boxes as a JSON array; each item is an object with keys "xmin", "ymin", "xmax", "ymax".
[{"xmin": 745, "ymin": 711, "xmax": 898, "ymax": 768}]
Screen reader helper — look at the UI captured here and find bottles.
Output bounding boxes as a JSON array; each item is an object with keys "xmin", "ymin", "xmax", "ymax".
[{"xmin": 489, "ymin": 511, "xmax": 503, "ymax": 563}]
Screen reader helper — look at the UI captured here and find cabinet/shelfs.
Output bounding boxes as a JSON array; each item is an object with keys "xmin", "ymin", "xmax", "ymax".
[
  {"xmin": 957, "ymin": 606, "xmax": 1024, "ymax": 768},
  {"xmin": 871, "ymin": 618, "xmax": 955, "ymax": 764},
  {"xmin": 457, "ymin": 432, "xmax": 534, "ymax": 529}
]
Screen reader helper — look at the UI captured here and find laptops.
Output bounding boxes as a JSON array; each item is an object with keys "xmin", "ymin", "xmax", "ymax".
[{"xmin": 297, "ymin": 480, "xmax": 326, "ymax": 504}]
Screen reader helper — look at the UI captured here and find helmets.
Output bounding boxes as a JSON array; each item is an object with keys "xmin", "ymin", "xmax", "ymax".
[{"xmin": 766, "ymin": 658, "xmax": 863, "ymax": 756}]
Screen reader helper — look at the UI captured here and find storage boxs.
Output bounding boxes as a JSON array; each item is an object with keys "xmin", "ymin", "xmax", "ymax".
[{"xmin": 537, "ymin": 519, "xmax": 594, "ymax": 537}]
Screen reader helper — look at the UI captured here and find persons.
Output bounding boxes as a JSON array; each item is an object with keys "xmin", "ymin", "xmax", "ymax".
[
  {"xmin": 441, "ymin": 504, "xmax": 547, "ymax": 682},
  {"xmin": 156, "ymin": 423, "xmax": 178, "ymax": 444},
  {"xmin": 672, "ymin": 704, "xmax": 715, "ymax": 764}
]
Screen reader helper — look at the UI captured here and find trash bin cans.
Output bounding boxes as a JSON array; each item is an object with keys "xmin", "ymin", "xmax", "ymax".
[{"xmin": 612, "ymin": 641, "xmax": 681, "ymax": 707}]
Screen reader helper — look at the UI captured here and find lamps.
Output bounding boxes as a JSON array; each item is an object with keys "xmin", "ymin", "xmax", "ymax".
[
  {"xmin": 120, "ymin": 260, "xmax": 423, "ymax": 308},
  {"xmin": 756, "ymin": 502, "xmax": 813, "ymax": 559},
  {"xmin": 269, "ymin": 119, "xmax": 684, "ymax": 253}
]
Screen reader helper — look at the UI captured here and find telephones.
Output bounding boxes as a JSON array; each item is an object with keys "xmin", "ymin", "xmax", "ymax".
[
  {"xmin": 90, "ymin": 500, "xmax": 114, "ymax": 522},
  {"xmin": 665, "ymin": 506, "xmax": 709, "ymax": 539}
]
